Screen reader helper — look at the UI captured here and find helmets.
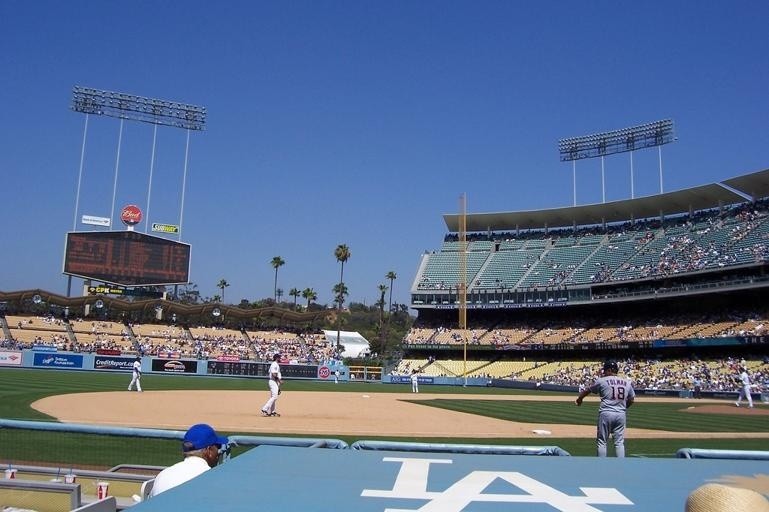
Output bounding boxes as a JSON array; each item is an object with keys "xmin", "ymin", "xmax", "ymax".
[{"xmin": 600, "ymin": 361, "xmax": 619, "ymax": 370}]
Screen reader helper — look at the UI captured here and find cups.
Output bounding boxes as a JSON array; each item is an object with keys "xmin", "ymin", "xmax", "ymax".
[
  {"xmin": 65, "ymin": 475, "xmax": 76, "ymax": 485},
  {"xmin": 5, "ymin": 469, "xmax": 17, "ymax": 480},
  {"xmin": 97, "ymin": 482, "xmax": 109, "ymax": 500}
]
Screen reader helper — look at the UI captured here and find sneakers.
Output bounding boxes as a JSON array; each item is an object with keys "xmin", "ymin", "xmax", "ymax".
[{"xmin": 260, "ymin": 409, "xmax": 281, "ymax": 417}]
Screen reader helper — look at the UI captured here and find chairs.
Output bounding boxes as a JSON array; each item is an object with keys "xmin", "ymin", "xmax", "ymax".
[
  {"xmin": 4, "ymin": 464, "xmax": 168, "ymax": 511},
  {"xmin": 414, "ymin": 208, "xmax": 768, "ymax": 314},
  {"xmin": 0, "ymin": 309, "xmax": 344, "ymax": 369},
  {"xmin": 402, "ymin": 315, "xmax": 768, "ymax": 349},
  {"xmin": 391, "ymin": 350, "xmax": 768, "ymax": 385}
]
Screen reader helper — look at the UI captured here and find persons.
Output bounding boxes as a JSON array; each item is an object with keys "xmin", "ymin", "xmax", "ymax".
[
  {"xmin": 370, "ymin": 372, "xmax": 376, "ymax": 380},
  {"xmin": 0, "ymin": 289, "xmax": 344, "ymax": 365},
  {"xmin": 383, "ymin": 194, "xmax": 769, "ymax": 394},
  {"xmin": 147, "ymin": 423, "xmax": 230, "ymax": 499},
  {"xmin": 127, "ymin": 356, "xmax": 142, "ymax": 391},
  {"xmin": 334, "ymin": 369, "xmax": 340, "ymax": 384},
  {"xmin": 731, "ymin": 364, "xmax": 753, "ymax": 409},
  {"xmin": 575, "ymin": 360, "xmax": 635, "ymax": 457},
  {"xmin": 260, "ymin": 353, "xmax": 283, "ymax": 418}
]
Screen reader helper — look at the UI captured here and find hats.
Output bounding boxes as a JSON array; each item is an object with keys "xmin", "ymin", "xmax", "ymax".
[
  {"xmin": 182, "ymin": 423, "xmax": 229, "ymax": 452},
  {"xmin": 273, "ymin": 354, "xmax": 282, "ymax": 358}
]
